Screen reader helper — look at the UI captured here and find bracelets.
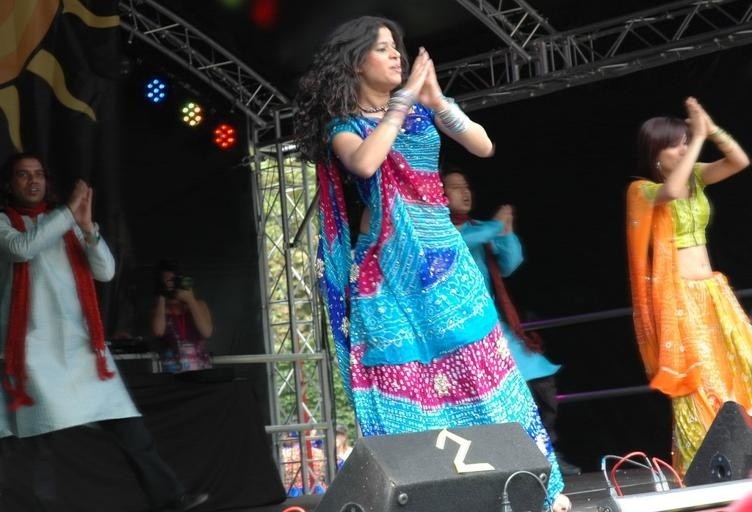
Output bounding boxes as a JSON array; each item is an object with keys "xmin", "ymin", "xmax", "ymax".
[
  {"xmin": 437, "ymin": 97, "xmax": 471, "ymax": 138},
  {"xmin": 379, "ymin": 86, "xmax": 417, "ymax": 129},
  {"xmin": 709, "ymin": 126, "xmax": 738, "ymax": 156}
]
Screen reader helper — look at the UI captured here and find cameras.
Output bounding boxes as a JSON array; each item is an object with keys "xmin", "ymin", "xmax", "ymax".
[{"xmin": 168, "ymin": 268, "xmax": 196, "ymax": 294}]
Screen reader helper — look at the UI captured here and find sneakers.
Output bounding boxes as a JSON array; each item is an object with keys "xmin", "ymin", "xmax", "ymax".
[{"xmin": 171, "ymin": 493, "xmax": 208, "ymax": 512}]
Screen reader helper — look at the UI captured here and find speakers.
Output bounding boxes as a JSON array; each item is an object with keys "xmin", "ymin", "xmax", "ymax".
[
  {"xmin": 682, "ymin": 399, "xmax": 752, "ymax": 486},
  {"xmin": 314, "ymin": 419, "xmax": 555, "ymax": 511}
]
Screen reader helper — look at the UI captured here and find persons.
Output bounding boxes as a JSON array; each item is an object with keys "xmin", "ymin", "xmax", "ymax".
[
  {"xmin": 287, "ymin": 16, "xmax": 577, "ymax": 511},
  {"xmin": 146, "ymin": 268, "xmax": 217, "ymax": 380},
  {"xmin": 440, "ymin": 167, "xmax": 583, "ymax": 481},
  {"xmin": 1, "ymin": 150, "xmax": 212, "ymax": 512},
  {"xmin": 621, "ymin": 94, "xmax": 751, "ymax": 485}
]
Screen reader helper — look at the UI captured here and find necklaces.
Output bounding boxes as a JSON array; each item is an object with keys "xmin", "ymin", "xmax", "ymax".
[{"xmin": 350, "ymin": 97, "xmax": 395, "ymax": 117}]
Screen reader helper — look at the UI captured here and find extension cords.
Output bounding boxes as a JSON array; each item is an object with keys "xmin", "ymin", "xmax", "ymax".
[{"xmin": 609, "ymin": 474, "xmax": 752, "ymax": 511}]
